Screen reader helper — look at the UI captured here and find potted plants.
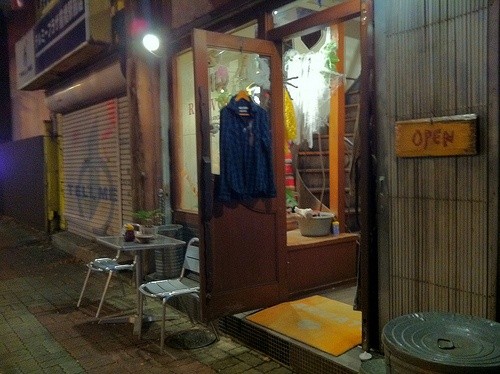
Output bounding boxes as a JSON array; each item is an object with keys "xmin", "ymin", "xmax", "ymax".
[{"xmin": 138, "ymin": 210, "xmax": 160, "ymax": 240}]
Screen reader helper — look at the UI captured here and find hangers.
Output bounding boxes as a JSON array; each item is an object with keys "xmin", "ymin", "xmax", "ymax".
[{"xmin": 232, "ymin": 79, "xmax": 253, "ymax": 117}]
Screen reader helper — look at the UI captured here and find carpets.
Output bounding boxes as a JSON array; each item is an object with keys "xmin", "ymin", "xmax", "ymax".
[{"xmin": 243, "ymin": 294, "xmax": 362, "ymax": 357}]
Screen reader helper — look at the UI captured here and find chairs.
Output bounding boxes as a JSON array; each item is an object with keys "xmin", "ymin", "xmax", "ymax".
[
  {"xmin": 77, "ymin": 223, "xmax": 142, "ymax": 318},
  {"xmin": 138, "ymin": 237, "xmax": 220, "ymax": 357}
]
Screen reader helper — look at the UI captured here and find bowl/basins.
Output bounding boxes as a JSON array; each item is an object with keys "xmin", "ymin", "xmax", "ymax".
[
  {"xmin": 135, "ymin": 235, "xmax": 152, "ymax": 243},
  {"xmin": 295, "ymin": 211, "xmax": 335, "ymax": 236}
]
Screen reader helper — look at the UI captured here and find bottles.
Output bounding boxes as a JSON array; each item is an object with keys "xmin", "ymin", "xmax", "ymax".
[
  {"xmin": 332, "ymin": 221, "xmax": 339, "ymax": 235},
  {"xmin": 124, "ymin": 224, "xmax": 135, "ymax": 241}
]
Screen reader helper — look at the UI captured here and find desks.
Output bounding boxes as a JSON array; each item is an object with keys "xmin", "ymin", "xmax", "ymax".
[{"xmin": 93, "ymin": 234, "xmax": 186, "ymax": 340}]
